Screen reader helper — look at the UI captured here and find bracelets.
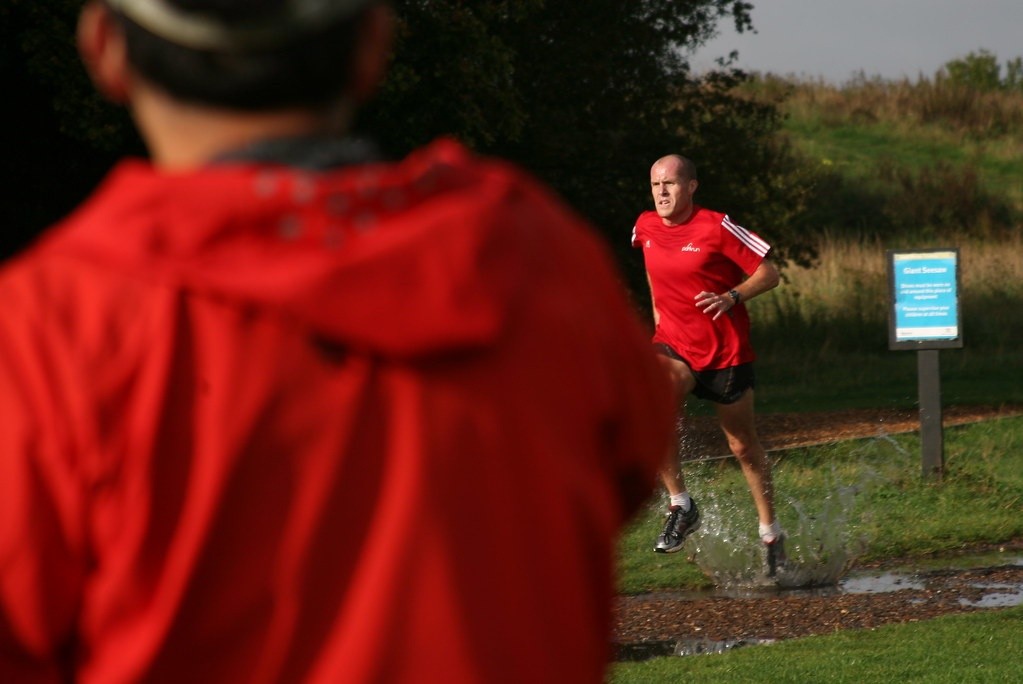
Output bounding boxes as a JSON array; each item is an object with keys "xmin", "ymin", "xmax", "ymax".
[{"xmin": 728, "ymin": 290, "xmax": 740, "ymax": 306}]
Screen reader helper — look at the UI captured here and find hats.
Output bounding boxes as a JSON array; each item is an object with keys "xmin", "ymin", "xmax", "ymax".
[{"xmin": 105, "ymin": 0, "xmax": 359, "ymax": 52}]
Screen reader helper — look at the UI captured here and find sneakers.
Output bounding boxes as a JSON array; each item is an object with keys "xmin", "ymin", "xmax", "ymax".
[
  {"xmin": 759, "ymin": 533, "xmax": 786, "ymax": 579},
  {"xmin": 653, "ymin": 497, "xmax": 701, "ymax": 553}
]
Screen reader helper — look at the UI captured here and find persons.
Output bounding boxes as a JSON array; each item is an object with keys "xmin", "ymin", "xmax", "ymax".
[
  {"xmin": 0, "ymin": 0, "xmax": 675, "ymax": 682},
  {"xmin": 631, "ymin": 153, "xmax": 788, "ymax": 578}
]
